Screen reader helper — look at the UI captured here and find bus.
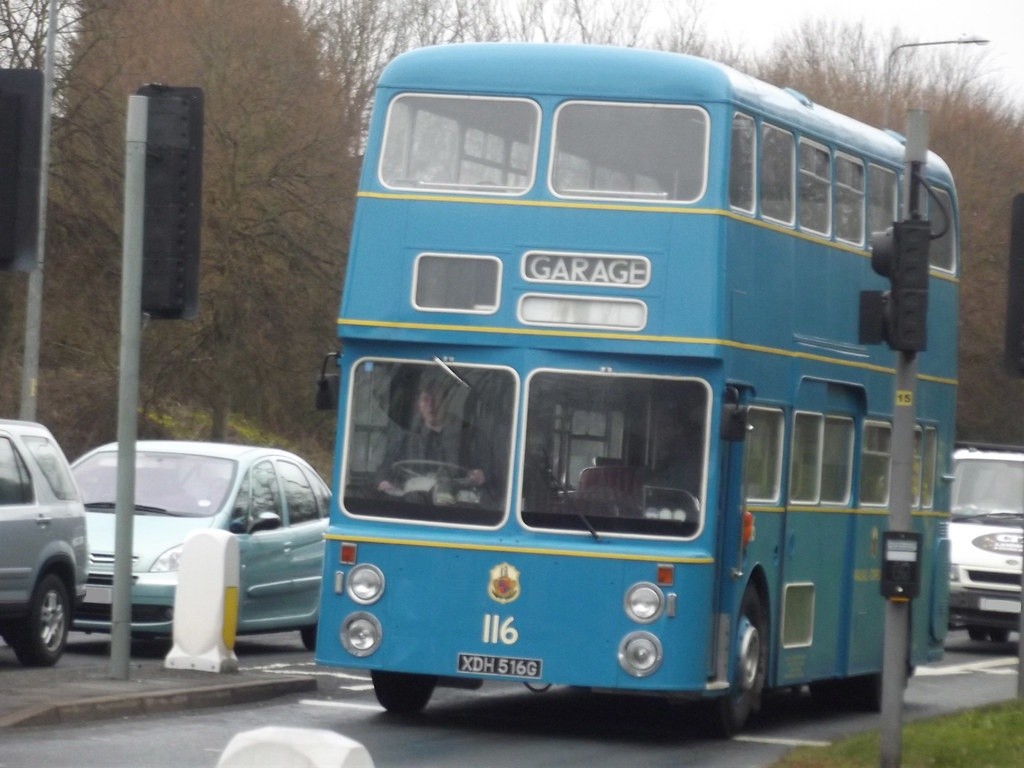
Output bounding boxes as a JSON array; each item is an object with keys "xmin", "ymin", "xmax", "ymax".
[{"xmin": 314, "ymin": 40, "xmax": 961, "ymax": 741}]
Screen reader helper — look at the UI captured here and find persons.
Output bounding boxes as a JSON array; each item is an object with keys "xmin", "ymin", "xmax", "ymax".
[
  {"xmin": 616, "ymin": 404, "xmax": 699, "ymax": 511},
  {"xmin": 370, "ymin": 380, "xmax": 500, "ymax": 504}
]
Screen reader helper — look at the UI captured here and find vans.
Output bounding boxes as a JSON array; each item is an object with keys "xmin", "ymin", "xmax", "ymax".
[{"xmin": 0, "ymin": 419, "xmax": 90, "ymax": 668}]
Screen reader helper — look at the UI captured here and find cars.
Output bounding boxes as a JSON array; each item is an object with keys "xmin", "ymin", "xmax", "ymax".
[
  {"xmin": 949, "ymin": 438, "xmax": 1024, "ymax": 645},
  {"xmin": 71, "ymin": 440, "xmax": 332, "ymax": 650}
]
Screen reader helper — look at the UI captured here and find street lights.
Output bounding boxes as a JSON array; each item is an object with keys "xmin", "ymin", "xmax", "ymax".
[{"xmin": 882, "ymin": 34, "xmax": 991, "ymax": 124}]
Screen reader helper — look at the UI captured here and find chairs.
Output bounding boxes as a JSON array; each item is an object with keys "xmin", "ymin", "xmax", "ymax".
[{"xmin": 582, "ymin": 466, "xmax": 642, "ymax": 517}]
